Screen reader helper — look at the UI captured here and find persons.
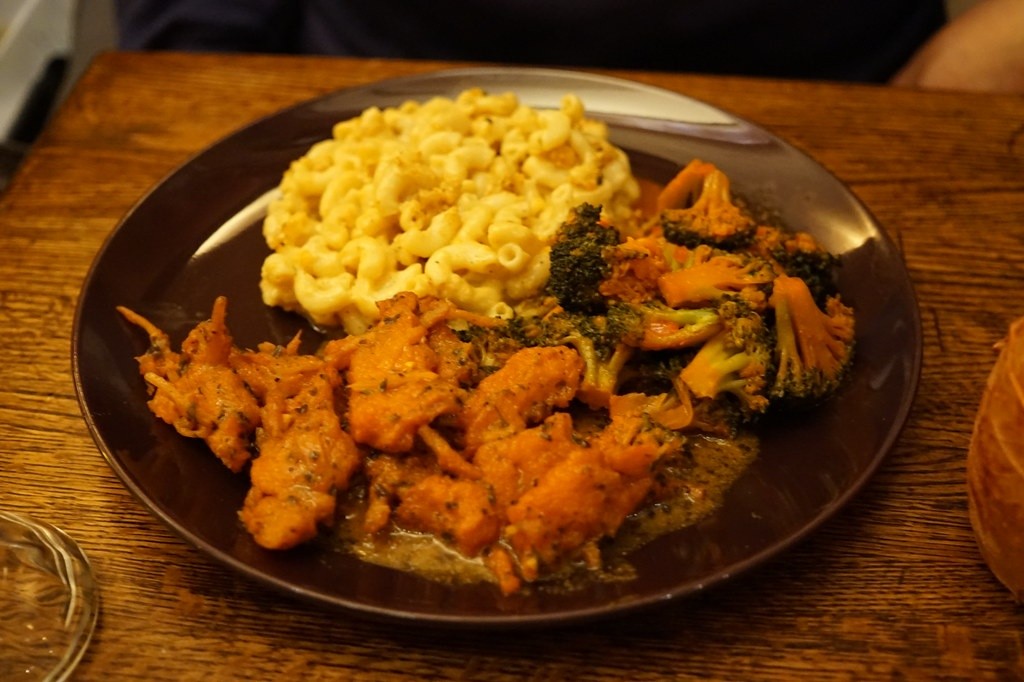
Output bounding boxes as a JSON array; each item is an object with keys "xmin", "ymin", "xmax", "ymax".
[{"xmin": 52, "ymin": 0, "xmax": 1024, "ymax": 116}]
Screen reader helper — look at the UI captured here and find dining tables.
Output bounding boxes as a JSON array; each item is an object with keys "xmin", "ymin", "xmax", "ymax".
[{"xmin": 0, "ymin": 48, "xmax": 1024, "ymax": 682}]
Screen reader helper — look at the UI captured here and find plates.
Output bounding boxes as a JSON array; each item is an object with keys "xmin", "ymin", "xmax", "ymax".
[
  {"xmin": 0, "ymin": 506, "xmax": 99, "ymax": 682},
  {"xmin": 71, "ymin": 67, "xmax": 923, "ymax": 629}
]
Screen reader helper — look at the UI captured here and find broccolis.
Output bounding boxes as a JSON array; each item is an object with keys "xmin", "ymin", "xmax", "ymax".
[{"xmin": 462, "ymin": 172, "xmax": 859, "ymax": 439}]
